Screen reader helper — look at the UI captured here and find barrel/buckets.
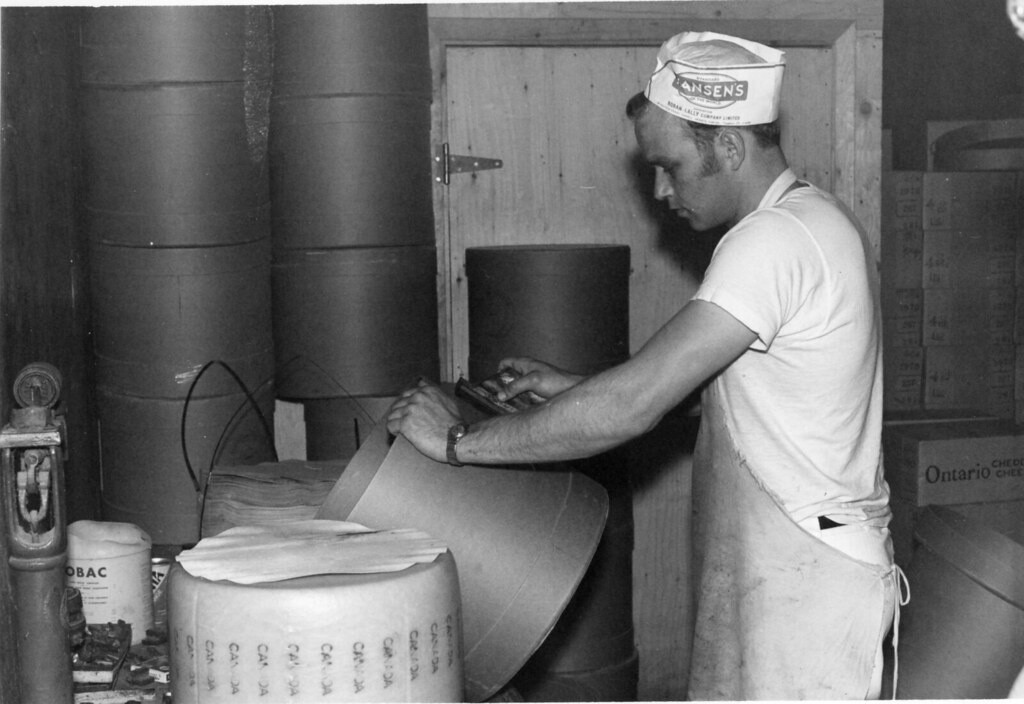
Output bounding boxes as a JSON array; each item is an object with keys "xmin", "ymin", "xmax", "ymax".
[{"xmin": 884, "ymin": 504, "xmax": 1024, "ymax": 700}]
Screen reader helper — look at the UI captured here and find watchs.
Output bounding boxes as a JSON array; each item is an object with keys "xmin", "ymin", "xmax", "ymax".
[{"xmin": 446, "ymin": 423, "xmax": 470, "ymax": 466}]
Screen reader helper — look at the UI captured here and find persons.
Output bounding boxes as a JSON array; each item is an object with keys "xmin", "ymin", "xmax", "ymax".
[{"xmin": 386, "ymin": 30, "xmax": 911, "ymax": 701}]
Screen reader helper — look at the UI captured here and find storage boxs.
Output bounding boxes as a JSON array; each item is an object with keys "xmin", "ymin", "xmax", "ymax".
[{"xmin": 884, "ymin": 166, "xmax": 1022, "ymax": 572}]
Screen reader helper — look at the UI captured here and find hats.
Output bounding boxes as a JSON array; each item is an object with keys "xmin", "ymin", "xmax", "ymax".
[{"xmin": 643, "ymin": 31, "xmax": 786, "ymax": 126}]
